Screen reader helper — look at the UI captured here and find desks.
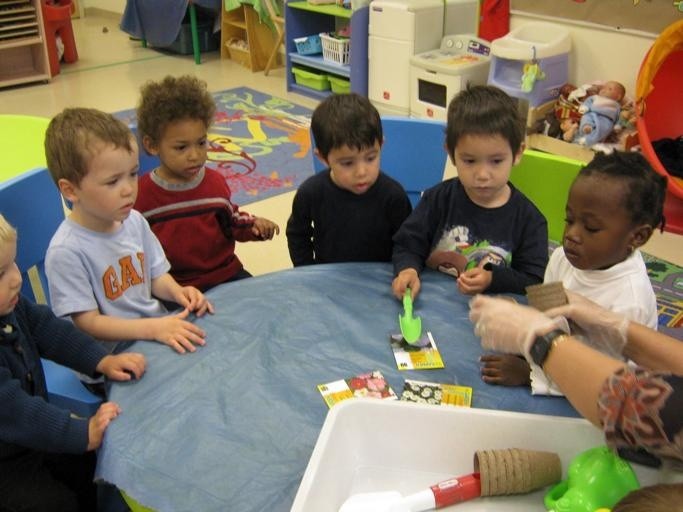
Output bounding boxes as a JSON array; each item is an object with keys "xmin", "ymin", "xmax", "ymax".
[{"xmin": 92, "ymin": 262, "xmax": 683, "ymax": 512}]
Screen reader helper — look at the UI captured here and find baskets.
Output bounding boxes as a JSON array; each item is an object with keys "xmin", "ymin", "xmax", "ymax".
[{"xmin": 319, "ymin": 32, "xmax": 350, "ymax": 65}]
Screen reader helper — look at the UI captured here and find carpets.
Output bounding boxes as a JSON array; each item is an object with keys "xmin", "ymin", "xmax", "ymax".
[
  {"xmin": 638, "ymin": 248, "xmax": 683, "ymax": 340},
  {"xmin": 95, "ymin": 86, "xmax": 327, "ymax": 207}
]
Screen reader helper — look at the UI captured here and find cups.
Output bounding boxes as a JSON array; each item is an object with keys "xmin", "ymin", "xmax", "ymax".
[{"xmin": 474, "ymin": 445, "xmax": 563, "ymax": 500}]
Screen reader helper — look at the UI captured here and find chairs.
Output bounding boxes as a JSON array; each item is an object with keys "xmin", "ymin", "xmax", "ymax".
[
  {"xmin": 0, "ymin": 169, "xmax": 108, "ymax": 414},
  {"xmin": 309, "ymin": 118, "xmax": 450, "ymax": 208},
  {"xmin": 509, "ymin": 151, "xmax": 588, "ymax": 247},
  {"xmin": 128, "ymin": 127, "xmax": 167, "ymax": 176}
]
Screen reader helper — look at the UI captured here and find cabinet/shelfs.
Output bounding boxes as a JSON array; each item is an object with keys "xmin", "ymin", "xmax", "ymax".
[
  {"xmin": 0, "ymin": 0, "xmax": 52, "ymax": 90},
  {"xmin": 221, "ymin": 1, "xmax": 280, "ymax": 70},
  {"xmin": 286, "ymin": 1, "xmax": 369, "ymax": 106}
]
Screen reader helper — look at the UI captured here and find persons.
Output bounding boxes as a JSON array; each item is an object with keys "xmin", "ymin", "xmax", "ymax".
[
  {"xmin": 468, "ymin": 288, "xmax": 683, "ymax": 473},
  {"xmin": 0, "ymin": 213, "xmax": 147, "ymax": 512},
  {"xmin": 285, "ymin": 94, "xmax": 413, "ymax": 267},
  {"xmin": 44, "ymin": 108, "xmax": 215, "ymax": 399},
  {"xmin": 132, "ymin": 76, "xmax": 280, "ymax": 312},
  {"xmin": 391, "ymin": 81, "xmax": 549, "ymax": 301},
  {"xmin": 477, "ymin": 148, "xmax": 668, "ymax": 397},
  {"xmin": 611, "ymin": 484, "xmax": 683, "ymax": 512},
  {"xmin": 578, "ymin": 80, "xmax": 625, "ymax": 147}
]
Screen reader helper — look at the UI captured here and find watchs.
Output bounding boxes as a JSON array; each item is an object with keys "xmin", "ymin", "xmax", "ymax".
[{"xmin": 530, "ymin": 328, "xmax": 567, "ymax": 368}]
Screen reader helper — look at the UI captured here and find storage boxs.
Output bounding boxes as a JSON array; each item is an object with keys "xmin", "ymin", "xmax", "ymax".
[
  {"xmin": 291, "ymin": 398, "xmax": 608, "ymax": 512},
  {"xmin": 150, "ymin": 18, "xmax": 213, "ymax": 55},
  {"xmin": 527, "ymin": 97, "xmax": 642, "ymax": 161}
]
